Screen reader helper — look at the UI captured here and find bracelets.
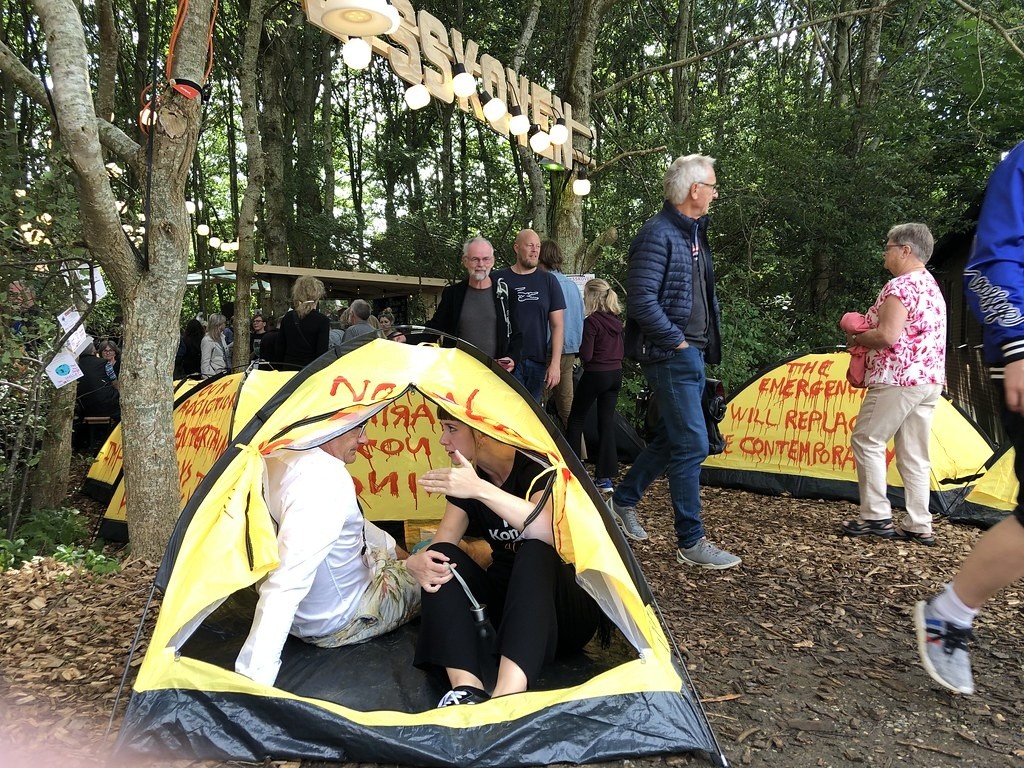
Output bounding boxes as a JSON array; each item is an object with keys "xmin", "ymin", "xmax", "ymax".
[{"xmin": 852, "ymin": 334, "xmax": 860, "ymax": 346}]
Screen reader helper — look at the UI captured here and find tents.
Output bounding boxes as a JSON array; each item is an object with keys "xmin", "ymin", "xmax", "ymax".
[
  {"xmin": 87, "ymin": 358, "xmax": 306, "ymax": 550},
  {"xmin": 100, "ymin": 324, "xmax": 728, "ymax": 765},
  {"xmin": 700, "ymin": 344, "xmax": 1020, "ymax": 529},
  {"xmin": 69, "ymin": 372, "xmax": 213, "ymax": 502}
]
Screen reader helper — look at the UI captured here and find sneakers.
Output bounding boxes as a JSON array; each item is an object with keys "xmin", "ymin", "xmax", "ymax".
[
  {"xmin": 606, "ymin": 496, "xmax": 650, "ymax": 542},
  {"xmin": 594, "ymin": 478, "xmax": 614, "ymax": 494},
  {"xmin": 677, "ymin": 538, "xmax": 742, "ymax": 570},
  {"xmin": 438, "ymin": 685, "xmax": 491, "ymax": 708},
  {"xmin": 912, "ymin": 598, "xmax": 974, "ymax": 695}
]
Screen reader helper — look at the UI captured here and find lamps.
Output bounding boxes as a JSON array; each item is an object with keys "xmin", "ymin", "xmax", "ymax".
[{"xmin": 315, "ymin": 0, "xmax": 399, "ymax": 37}]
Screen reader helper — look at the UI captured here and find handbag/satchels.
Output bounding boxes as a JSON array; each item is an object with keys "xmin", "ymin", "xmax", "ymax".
[{"xmin": 635, "ymin": 377, "xmax": 727, "ymax": 455}]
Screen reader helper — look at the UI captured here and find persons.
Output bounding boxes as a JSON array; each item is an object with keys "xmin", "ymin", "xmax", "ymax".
[
  {"xmin": 567, "ymin": 279, "xmax": 623, "ymax": 492},
  {"xmin": 10, "ymin": 274, "xmax": 397, "ymax": 429},
  {"xmin": 539, "ymin": 241, "xmax": 587, "ymax": 465},
  {"xmin": 915, "ymin": 140, "xmax": 1024, "ymax": 696},
  {"xmin": 489, "ymin": 229, "xmax": 568, "ymax": 406},
  {"xmin": 841, "ymin": 223, "xmax": 947, "ymax": 546},
  {"xmin": 235, "ymin": 418, "xmax": 494, "ymax": 688},
  {"xmin": 407, "ymin": 404, "xmax": 616, "ymax": 709},
  {"xmin": 605, "ymin": 153, "xmax": 743, "ymax": 570},
  {"xmin": 391, "ymin": 236, "xmax": 522, "ymax": 372}
]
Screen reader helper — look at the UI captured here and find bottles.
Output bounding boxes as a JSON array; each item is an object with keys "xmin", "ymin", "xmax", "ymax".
[
  {"xmin": 105, "ymin": 363, "xmax": 117, "ymax": 381},
  {"xmin": 471, "ymin": 604, "xmax": 496, "ymax": 693}
]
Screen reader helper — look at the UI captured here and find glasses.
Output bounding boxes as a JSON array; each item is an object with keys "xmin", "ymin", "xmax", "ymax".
[
  {"xmin": 253, "ymin": 320, "xmax": 264, "ymax": 323},
  {"xmin": 883, "ymin": 245, "xmax": 905, "ymax": 250},
  {"xmin": 356, "ymin": 421, "xmax": 368, "ymax": 437},
  {"xmin": 104, "ymin": 348, "xmax": 115, "ymax": 355},
  {"xmin": 698, "ymin": 182, "xmax": 721, "ymax": 191}
]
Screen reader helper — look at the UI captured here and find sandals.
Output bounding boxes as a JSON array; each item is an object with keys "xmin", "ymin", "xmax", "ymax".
[
  {"xmin": 891, "ymin": 527, "xmax": 934, "ymax": 547},
  {"xmin": 842, "ymin": 519, "xmax": 895, "ymax": 538}
]
